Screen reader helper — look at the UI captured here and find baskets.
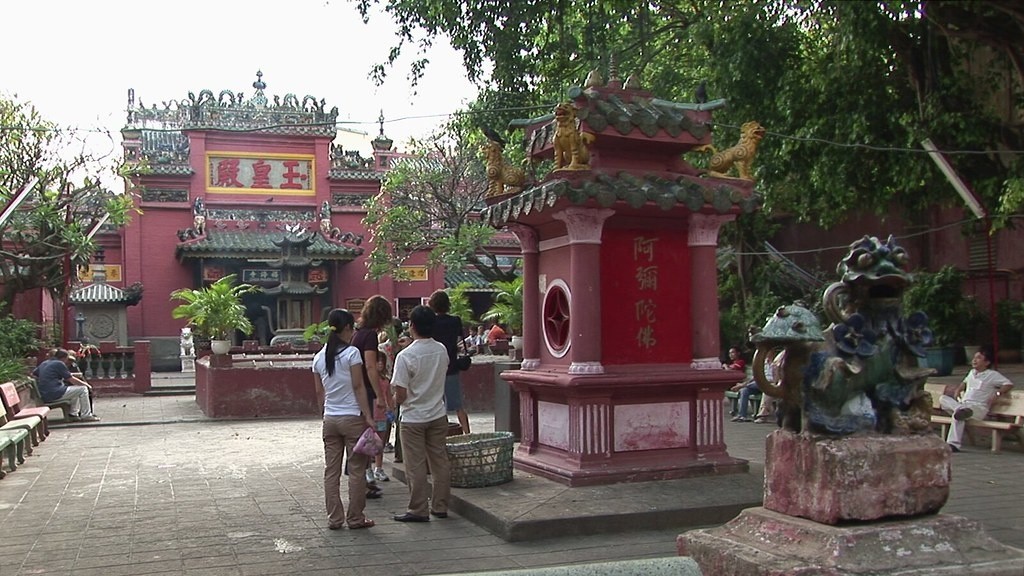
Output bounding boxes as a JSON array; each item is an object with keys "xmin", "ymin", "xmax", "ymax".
[{"xmin": 446, "ymin": 432, "xmax": 514, "ymax": 488}]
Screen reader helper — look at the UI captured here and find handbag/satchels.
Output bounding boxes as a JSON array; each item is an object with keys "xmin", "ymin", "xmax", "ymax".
[
  {"xmin": 458, "ymin": 354, "xmax": 472, "ymax": 370},
  {"xmin": 352, "ymin": 426, "xmax": 384, "ymax": 456}
]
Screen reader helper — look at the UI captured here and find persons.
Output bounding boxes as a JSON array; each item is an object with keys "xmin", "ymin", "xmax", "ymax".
[
  {"xmin": 457, "ymin": 318, "xmax": 505, "ymax": 356},
  {"xmin": 390, "ymin": 305, "xmax": 451, "ymax": 523},
  {"xmin": 349, "ymin": 295, "xmax": 410, "ymax": 499},
  {"xmin": 429, "ymin": 292, "xmax": 470, "ymax": 434},
  {"xmin": 939, "ymin": 348, "xmax": 1014, "ymax": 452},
  {"xmin": 312, "ymin": 309, "xmax": 379, "ymax": 530},
  {"xmin": 31, "ymin": 349, "xmax": 101, "ymax": 421},
  {"xmin": 723, "ymin": 346, "xmax": 774, "ymax": 423}
]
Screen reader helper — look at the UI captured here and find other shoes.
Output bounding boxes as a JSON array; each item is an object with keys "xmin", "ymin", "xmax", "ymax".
[
  {"xmin": 730, "ymin": 416, "xmax": 745, "ymax": 422},
  {"xmin": 350, "ymin": 518, "xmax": 374, "ymax": 528},
  {"xmin": 66, "ymin": 414, "xmax": 101, "ymax": 422},
  {"xmin": 395, "ymin": 513, "xmax": 429, "ymax": 521},
  {"xmin": 364, "ymin": 483, "xmax": 382, "ymax": 498},
  {"xmin": 753, "ymin": 412, "xmax": 771, "ymax": 424},
  {"xmin": 954, "ymin": 406, "xmax": 972, "ymax": 422},
  {"xmin": 430, "ymin": 510, "xmax": 448, "ymax": 518}
]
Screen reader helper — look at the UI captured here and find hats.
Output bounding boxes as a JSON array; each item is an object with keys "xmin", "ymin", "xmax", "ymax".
[{"xmin": 68, "ymin": 350, "xmax": 77, "ymax": 361}]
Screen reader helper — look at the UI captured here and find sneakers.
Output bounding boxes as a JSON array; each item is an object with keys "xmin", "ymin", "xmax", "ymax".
[
  {"xmin": 366, "ymin": 468, "xmax": 375, "ymax": 483},
  {"xmin": 374, "ymin": 465, "xmax": 389, "ymax": 481}
]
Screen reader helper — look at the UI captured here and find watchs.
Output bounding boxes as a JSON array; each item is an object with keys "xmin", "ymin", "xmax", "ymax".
[{"xmin": 996, "ymin": 391, "xmax": 1001, "ymax": 397}]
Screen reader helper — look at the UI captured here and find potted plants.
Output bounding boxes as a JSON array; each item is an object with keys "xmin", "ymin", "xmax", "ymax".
[
  {"xmin": 480, "ymin": 277, "xmax": 523, "ymax": 348},
  {"xmin": 169, "ymin": 273, "xmax": 265, "ymax": 355}
]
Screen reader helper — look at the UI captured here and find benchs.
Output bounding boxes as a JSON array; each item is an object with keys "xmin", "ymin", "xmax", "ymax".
[
  {"xmin": 722, "ymin": 369, "xmax": 1024, "ymax": 454},
  {"xmin": 31, "ymin": 379, "xmax": 72, "ymax": 422}
]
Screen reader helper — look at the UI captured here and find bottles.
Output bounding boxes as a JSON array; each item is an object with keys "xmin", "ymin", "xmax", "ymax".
[{"xmin": 384, "ymin": 406, "xmax": 396, "ymax": 424}]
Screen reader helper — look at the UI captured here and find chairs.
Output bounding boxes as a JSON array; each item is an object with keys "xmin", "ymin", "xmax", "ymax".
[{"xmin": 0, "ymin": 382, "xmax": 51, "ymax": 480}]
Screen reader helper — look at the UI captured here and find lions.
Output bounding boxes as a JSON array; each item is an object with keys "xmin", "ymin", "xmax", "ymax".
[{"xmin": 179, "ymin": 327, "xmax": 195, "ymax": 356}]
